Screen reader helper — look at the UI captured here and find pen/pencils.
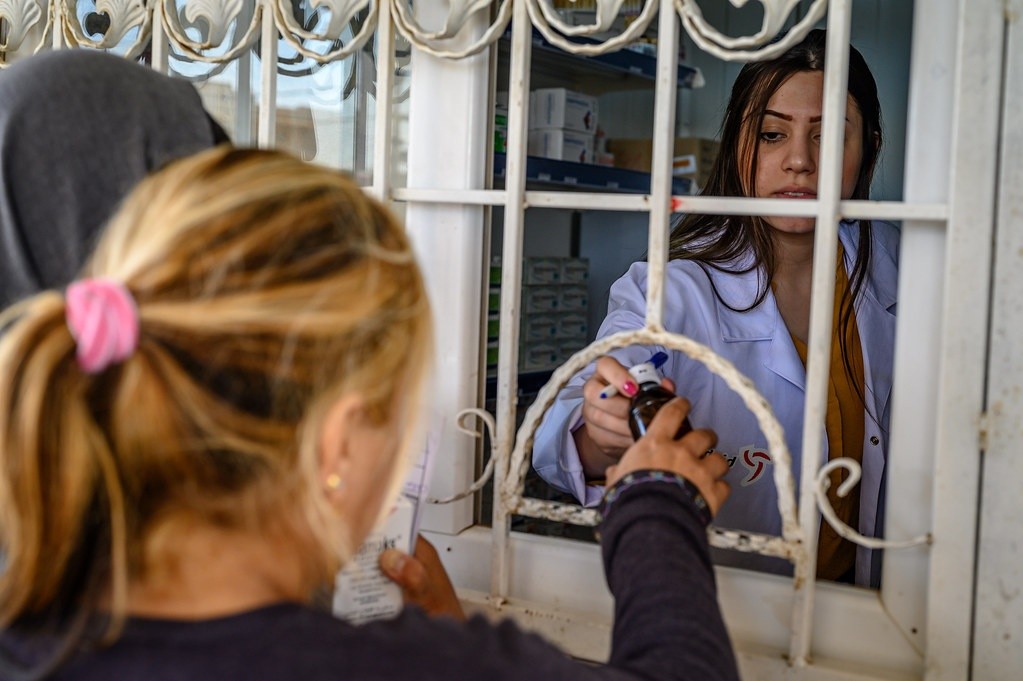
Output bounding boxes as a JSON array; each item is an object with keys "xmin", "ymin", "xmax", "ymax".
[{"xmin": 600, "ymin": 351, "xmax": 670, "ymax": 400}]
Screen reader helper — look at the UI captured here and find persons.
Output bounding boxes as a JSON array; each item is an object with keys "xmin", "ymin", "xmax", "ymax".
[
  {"xmin": 1, "ymin": 47, "xmax": 465, "ymax": 622},
  {"xmin": 0, "ymin": 144, "xmax": 741, "ymax": 680},
  {"xmin": 531, "ymin": 29, "xmax": 901, "ymax": 591}
]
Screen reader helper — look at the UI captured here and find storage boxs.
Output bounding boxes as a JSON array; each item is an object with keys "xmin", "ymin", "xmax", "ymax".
[
  {"xmin": 495, "ymin": 89, "xmax": 721, "ymax": 184},
  {"xmin": 486, "ymin": 257, "xmax": 589, "ymax": 370}
]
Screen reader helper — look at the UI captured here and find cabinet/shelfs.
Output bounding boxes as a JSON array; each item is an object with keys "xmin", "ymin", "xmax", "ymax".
[{"xmin": 485, "ymin": 21, "xmax": 706, "ymax": 407}]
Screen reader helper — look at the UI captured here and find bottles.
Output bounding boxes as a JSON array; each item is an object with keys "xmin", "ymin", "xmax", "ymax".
[{"xmin": 628, "ymin": 363, "xmax": 693, "ymax": 441}]
